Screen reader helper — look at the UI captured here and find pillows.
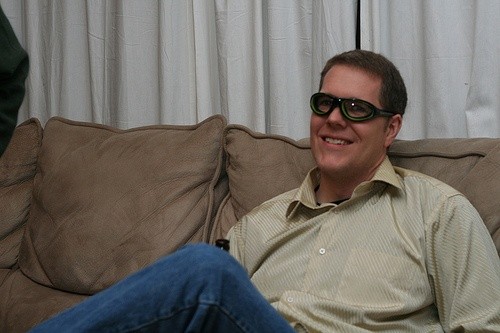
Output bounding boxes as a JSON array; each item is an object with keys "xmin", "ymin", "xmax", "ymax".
[
  {"xmin": 210, "ymin": 123, "xmax": 499, "ymax": 292},
  {"xmin": 0, "ymin": 117, "xmax": 42, "ymax": 268},
  {"xmin": 17, "ymin": 111, "xmax": 227, "ymax": 292}
]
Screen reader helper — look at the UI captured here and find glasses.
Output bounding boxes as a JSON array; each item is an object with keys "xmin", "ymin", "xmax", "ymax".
[{"xmin": 309, "ymin": 92, "xmax": 398, "ymax": 123}]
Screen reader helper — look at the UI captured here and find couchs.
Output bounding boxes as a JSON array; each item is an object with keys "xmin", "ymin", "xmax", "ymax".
[{"xmin": 0, "ymin": 272, "xmax": 97, "ymax": 333}]
